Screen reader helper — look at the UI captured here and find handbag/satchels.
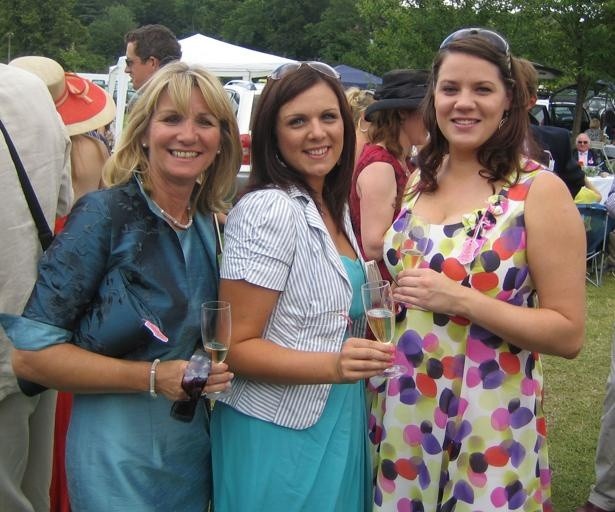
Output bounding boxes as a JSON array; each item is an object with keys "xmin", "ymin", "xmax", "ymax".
[{"xmin": 18, "ymin": 267, "xmax": 168, "ymax": 398}]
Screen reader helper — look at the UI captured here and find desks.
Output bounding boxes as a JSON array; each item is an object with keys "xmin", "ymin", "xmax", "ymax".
[{"xmin": 584, "ymin": 172, "xmax": 615, "ymax": 206}]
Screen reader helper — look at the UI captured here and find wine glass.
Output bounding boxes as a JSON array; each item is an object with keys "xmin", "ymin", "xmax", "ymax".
[
  {"xmin": 200, "ymin": 301, "xmax": 232, "ymax": 400},
  {"xmin": 360, "ymin": 280, "xmax": 408, "ymax": 380}
]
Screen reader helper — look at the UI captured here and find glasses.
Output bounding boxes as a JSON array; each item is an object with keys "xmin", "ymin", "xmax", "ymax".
[
  {"xmin": 439, "ymin": 28, "xmax": 513, "ymax": 79},
  {"xmin": 170, "ymin": 348, "xmax": 210, "ymax": 424},
  {"xmin": 268, "ymin": 61, "xmax": 341, "ymax": 80},
  {"xmin": 578, "ymin": 141, "xmax": 588, "ymax": 144},
  {"xmin": 125, "ymin": 55, "xmax": 143, "ymax": 65}
]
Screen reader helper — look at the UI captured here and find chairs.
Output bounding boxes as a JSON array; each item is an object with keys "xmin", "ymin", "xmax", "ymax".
[
  {"xmin": 591, "ymin": 141, "xmax": 615, "ymax": 173},
  {"xmin": 575, "ymin": 204, "xmax": 615, "ymax": 288}
]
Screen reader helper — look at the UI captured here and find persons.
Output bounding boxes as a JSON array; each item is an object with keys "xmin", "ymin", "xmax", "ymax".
[
  {"xmin": 0, "ymin": 56, "xmax": 395, "ymax": 512},
  {"xmin": 574, "ymin": 331, "xmax": 615, "ymax": 511},
  {"xmin": 585, "ymin": 115, "xmax": 611, "ymax": 144},
  {"xmin": 350, "ymin": 29, "xmax": 586, "ymax": 512},
  {"xmin": 124, "ymin": 24, "xmax": 182, "ymax": 91},
  {"xmin": 573, "ymin": 133, "xmax": 615, "ymax": 271}
]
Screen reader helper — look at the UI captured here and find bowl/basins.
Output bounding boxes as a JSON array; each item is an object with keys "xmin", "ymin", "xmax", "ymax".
[{"xmin": 582, "ymin": 165, "xmax": 601, "ymax": 177}]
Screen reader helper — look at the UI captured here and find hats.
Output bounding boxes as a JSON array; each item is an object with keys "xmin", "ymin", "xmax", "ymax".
[
  {"xmin": 8, "ymin": 55, "xmax": 117, "ymax": 137},
  {"xmin": 364, "ymin": 71, "xmax": 434, "ymax": 122}
]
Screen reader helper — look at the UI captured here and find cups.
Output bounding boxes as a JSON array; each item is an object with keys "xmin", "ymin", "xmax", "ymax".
[{"xmin": 391, "ymin": 213, "xmax": 432, "ymax": 271}]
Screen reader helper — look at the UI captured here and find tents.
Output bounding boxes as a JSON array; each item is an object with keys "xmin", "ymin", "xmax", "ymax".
[{"xmin": 107, "ymin": 33, "xmax": 302, "ymax": 154}]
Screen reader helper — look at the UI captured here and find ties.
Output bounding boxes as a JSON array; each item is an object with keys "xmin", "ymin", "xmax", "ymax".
[{"xmin": 581, "ymin": 153, "xmax": 585, "ymax": 165}]
